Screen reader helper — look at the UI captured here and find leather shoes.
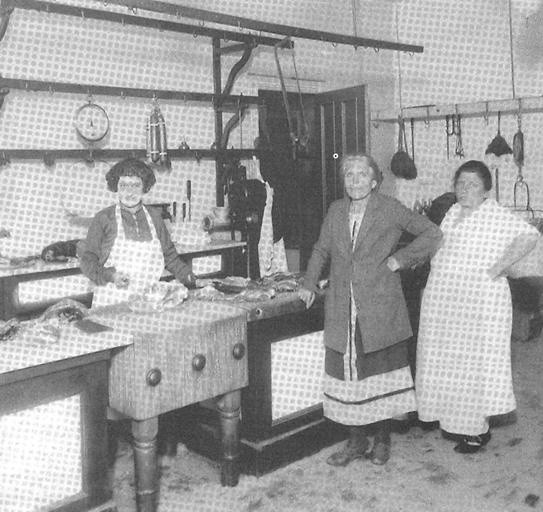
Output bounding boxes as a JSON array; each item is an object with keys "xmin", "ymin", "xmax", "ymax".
[
  {"xmin": 369, "ymin": 435, "xmax": 393, "ymax": 467},
  {"xmin": 326, "ymin": 435, "xmax": 368, "ymax": 467}
]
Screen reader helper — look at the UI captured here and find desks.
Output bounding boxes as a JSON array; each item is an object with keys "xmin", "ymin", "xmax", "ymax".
[
  {"xmin": 0, "ymin": 275, "xmax": 332, "ymax": 507},
  {"xmin": 2, "ymin": 238, "xmax": 247, "ymax": 318}
]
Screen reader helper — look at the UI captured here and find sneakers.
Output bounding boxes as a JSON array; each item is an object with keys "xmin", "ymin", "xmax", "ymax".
[{"xmin": 453, "ymin": 431, "xmax": 491, "ymax": 455}]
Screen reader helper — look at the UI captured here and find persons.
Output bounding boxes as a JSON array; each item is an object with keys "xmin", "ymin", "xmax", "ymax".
[
  {"xmin": 417, "ymin": 160, "xmax": 537, "ymax": 452},
  {"xmin": 298, "ymin": 154, "xmax": 442, "ymax": 466},
  {"xmin": 78, "ymin": 157, "xmax": 213, "ymax": 306}
]
